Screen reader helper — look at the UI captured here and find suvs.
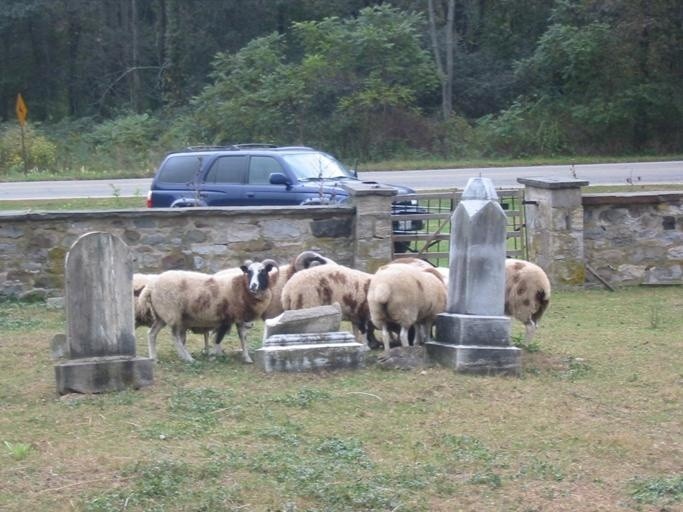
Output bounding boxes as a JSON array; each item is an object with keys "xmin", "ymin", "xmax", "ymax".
[{"xmin": 146, "ymin": 141, "xmax": 428, "ymax": 255}]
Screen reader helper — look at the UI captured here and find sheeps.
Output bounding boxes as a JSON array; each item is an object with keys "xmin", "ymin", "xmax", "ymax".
[
  {"xmin": 131, "ymin": 248, "xmax": 451, "ymax": 365},
  {"xmin": 505, "ymin": 256, "xmax": 552, "ymax": 348}
]
